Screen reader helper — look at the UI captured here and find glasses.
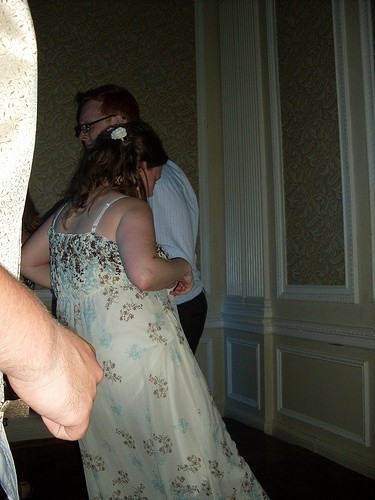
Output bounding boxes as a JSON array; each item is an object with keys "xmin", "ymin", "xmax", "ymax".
[{"xmin": 78, "ymin": 115, "xmax": 126, "ymax": 134}]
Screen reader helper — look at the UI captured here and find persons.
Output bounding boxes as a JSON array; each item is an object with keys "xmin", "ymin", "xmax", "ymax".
[
  {"xmin": 20, "ymin": 119, "xmax": 273, "ymax": 500},
  {"xmin": 17, "ymin": 196, "xmax": 45, "ymax": 292},
  {"xmin": 1, "ymin": 0, "xmax": 105, "ymax": 500},
  {"xmin": 75, "ymin": 81, "xmax": 209, "ymax": 361}
]
